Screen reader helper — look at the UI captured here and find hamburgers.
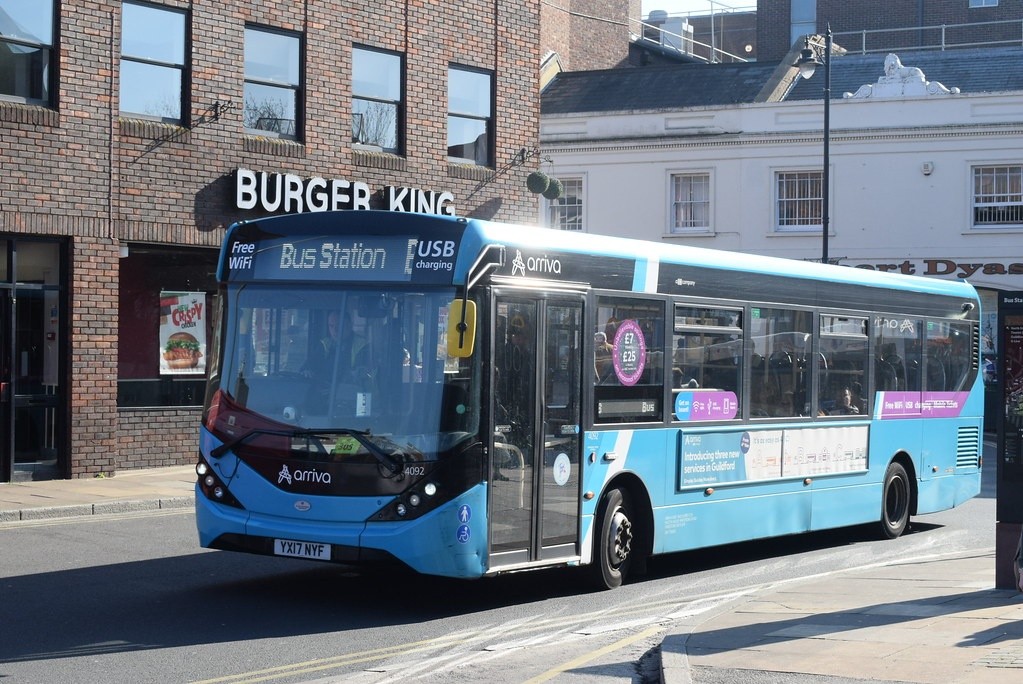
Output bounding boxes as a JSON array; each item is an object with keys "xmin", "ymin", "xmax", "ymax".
[{"xmin": 163, "ymin": 331, "xmax": 204, "ymax": 369}]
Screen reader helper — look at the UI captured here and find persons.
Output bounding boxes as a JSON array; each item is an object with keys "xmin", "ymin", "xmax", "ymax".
[
  {"xmin": 312, "ymin": 304, "xmax": 373, "ymax": 399},
  {"xmin": 402, "ymin": 349, "xmax": 421, "ymax": 384},
  {"xmin": 594, "ymin": 331, "xmax": 616, "ymax": 382},
  {"xmin": 493, "ymin": 314, "xmax": 532, "ymax": 481},
  {"xmin": 818, "ymin": 384, "xmax": 860, "ymax": 417},
  {"xmin": 676, "ymin": 330, "xmax": 782, "ymax": 416}
]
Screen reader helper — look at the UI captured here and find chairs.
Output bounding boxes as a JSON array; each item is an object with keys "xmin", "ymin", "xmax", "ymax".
[
  {"xmin": 686, "ymin": 348, "xmax": 954, "ymax": 418},
  {"xmin": 367, "ymin": 324, "xmax": 389, "ymax": 373}
]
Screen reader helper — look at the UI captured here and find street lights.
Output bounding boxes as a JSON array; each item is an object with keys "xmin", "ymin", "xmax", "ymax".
[{"xmin": 791, "ymin": 21, "xmax": 832, "ymax": 264}]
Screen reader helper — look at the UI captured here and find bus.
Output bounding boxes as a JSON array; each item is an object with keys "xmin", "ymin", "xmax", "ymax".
[{"xmin": 194, "ymin": 208, "xmax": 986, "ymax": 591}]
